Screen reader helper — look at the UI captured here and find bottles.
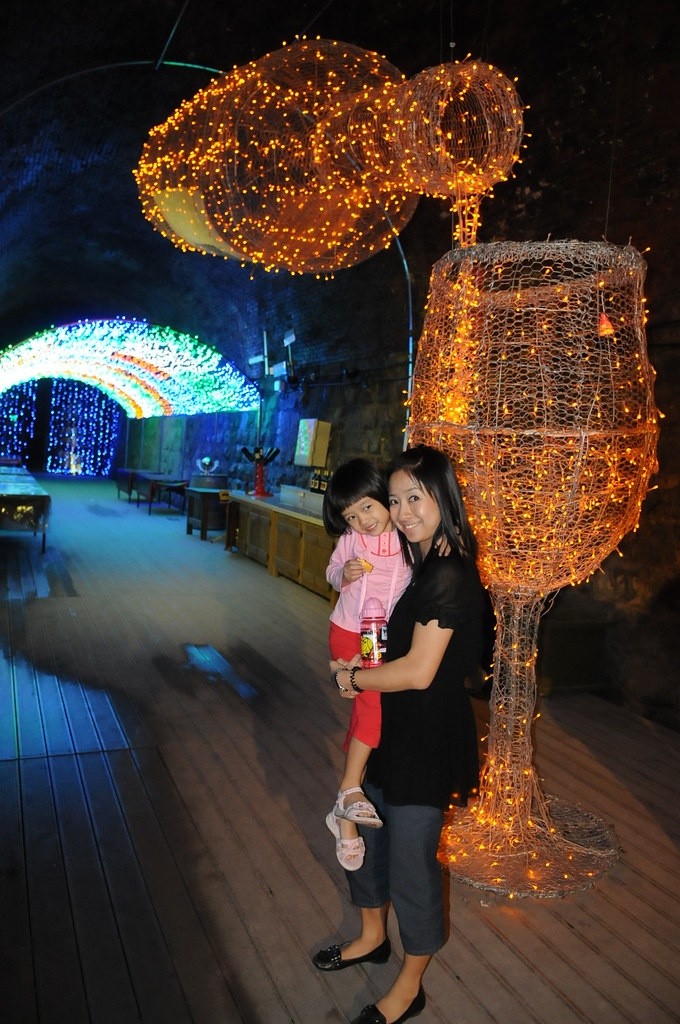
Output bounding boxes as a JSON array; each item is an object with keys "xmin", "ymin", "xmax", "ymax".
[
  {"xmin": 239, "ymin": 446, "xmax": 279, "ymax": 465},
  {"xmin": 139, "ymin": 43, "xmax": 523, "ymax": 277},
  {"xmin": 310, "ymin": 469, "xmax": 333, "ymax": 494}
]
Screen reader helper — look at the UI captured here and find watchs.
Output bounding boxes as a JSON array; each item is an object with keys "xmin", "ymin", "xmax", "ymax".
[{"xmin": 334, "ymin": 666, "xmax": 349, "ymax": 692}]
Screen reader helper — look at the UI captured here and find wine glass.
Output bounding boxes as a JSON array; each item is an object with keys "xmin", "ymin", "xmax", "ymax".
[{"xmin": 405, "ymin": 242, "xmax": 664, "ymax": 901}]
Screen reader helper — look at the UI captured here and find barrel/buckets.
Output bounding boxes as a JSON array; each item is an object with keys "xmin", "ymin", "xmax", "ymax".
[{"xmin": 191, "ymin": 472, "xmax": 230, "ymax": 532}]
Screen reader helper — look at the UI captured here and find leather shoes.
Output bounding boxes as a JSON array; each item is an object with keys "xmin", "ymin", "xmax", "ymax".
[
  {"xmin": 350, "ymin": 984, "xmax": 426, "ymax": 1024},
  {"xmin": 313, "ymin": 935, "xmax": 391, "ymax": 971}
]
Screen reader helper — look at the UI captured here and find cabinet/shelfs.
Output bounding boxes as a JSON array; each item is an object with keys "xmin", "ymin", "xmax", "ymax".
[{"xmin": 230, "ymin": 491, "xmax": 340, "ymax": 608}]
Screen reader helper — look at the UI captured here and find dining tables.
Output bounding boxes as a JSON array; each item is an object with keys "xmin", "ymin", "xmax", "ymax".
[
  {"xmin": 184, "ymin": 485, "xmax": 230, "ymax": 542},
  {"xmin": 116, "ymin": 466, "xmax": 163, "ymax": 504},
  {"xmin": 0, "ymin": 457, "xmax": 50, "ymax": 553},
  {"xmin": 136, "ymin": 473, "xmax": 189, "ymax": 517}
]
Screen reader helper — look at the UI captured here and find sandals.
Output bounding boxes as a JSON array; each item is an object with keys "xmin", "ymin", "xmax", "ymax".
[
  {"xmin": 334, "ymin": 787, "xmax": 384, "ymax": 829},
  {"xmin": 325, "ymin": 804, "xmax": 366, "ymax": 871}
]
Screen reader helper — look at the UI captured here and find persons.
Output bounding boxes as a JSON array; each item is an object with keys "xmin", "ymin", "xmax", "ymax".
[
  {"xmin": 312, "ymin": 441, "xmax": 480, "ymax": 1023},
  {"xmin": 323, "ymin": 457, "xmax": 466, "ymax": 870}
]
